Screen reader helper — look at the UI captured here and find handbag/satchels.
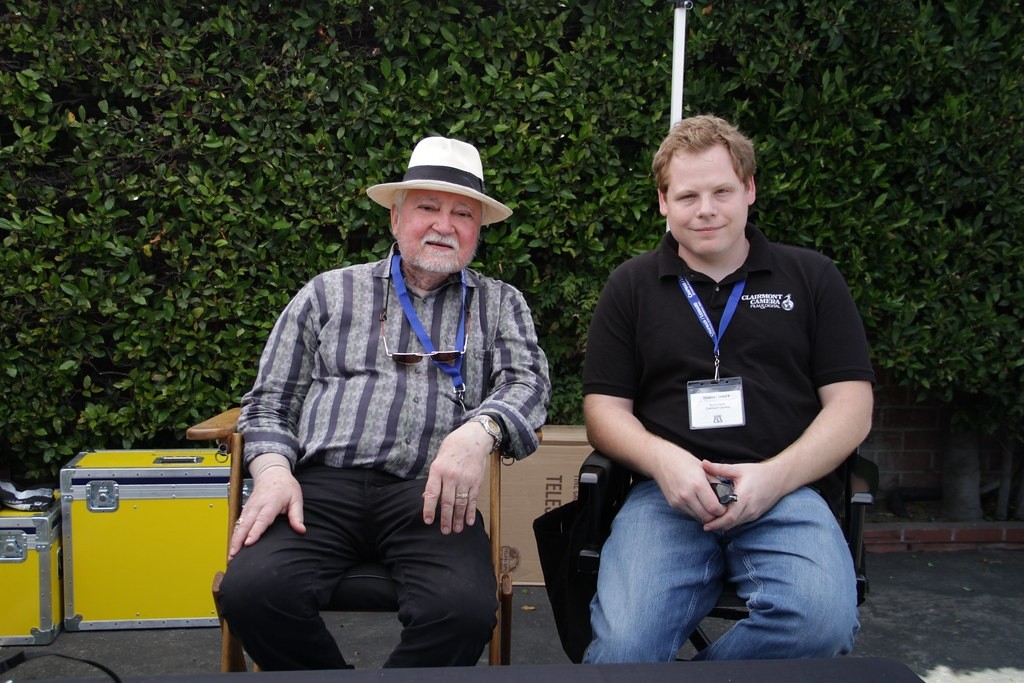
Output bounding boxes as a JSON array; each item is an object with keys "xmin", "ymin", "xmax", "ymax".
[{"xmin": 532, "ymin": 497, "xmax": 617, "ymax": 664}]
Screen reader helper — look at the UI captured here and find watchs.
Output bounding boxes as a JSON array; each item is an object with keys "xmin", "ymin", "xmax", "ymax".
[{"xmin": 468, "ymin": 416, "xmax": 503, "ymax": 455}]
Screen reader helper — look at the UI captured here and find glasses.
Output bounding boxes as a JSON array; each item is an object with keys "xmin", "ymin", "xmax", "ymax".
[{"xmin": 380, "ymin": 307, "xmax": 471, "ymax": 366}]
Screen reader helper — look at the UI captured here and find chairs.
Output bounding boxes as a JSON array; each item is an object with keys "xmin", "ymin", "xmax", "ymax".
[
  {"xmin": 534, "ymin": 446, "xmax": 879, "ymax": 664},
  {"xmin": 186, "ymin": 406, "xmax": 543, "ymax": 683}
]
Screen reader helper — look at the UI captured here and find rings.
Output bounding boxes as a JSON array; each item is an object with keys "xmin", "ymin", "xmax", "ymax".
[
  {"xmin": 456, "ymin": 494, "xmax": 469, "ymax": 499},
  {"xmin": 236, "ymin": 519, "xmax": 243, "ymax": 525}
]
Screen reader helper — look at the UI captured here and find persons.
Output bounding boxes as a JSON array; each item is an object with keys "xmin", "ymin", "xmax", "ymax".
[
  {"xmin": 219, "ymin": 136, "xmax": 549, "ymax": 671},
  {"xmin": 577, "ymin": 115, "xmax": 876, "ymax": 664}
]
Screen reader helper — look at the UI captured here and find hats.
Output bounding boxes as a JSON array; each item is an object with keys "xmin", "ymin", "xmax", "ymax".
[{"xmin": 366, "ymin": 137, "xmax": 514, "ymax": 227}]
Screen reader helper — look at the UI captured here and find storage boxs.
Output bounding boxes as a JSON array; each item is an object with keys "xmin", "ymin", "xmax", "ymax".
[
  {"xmin": 59, "ymin": 449, "xmax": 234, "ymax": 632},
  {"xmin": 0, "ymin": 504, "xmax": 65, "ymax": 648},
  {"xmin": 481, "ymin": 425, "xmax": 602, "ymax": 588}
]
